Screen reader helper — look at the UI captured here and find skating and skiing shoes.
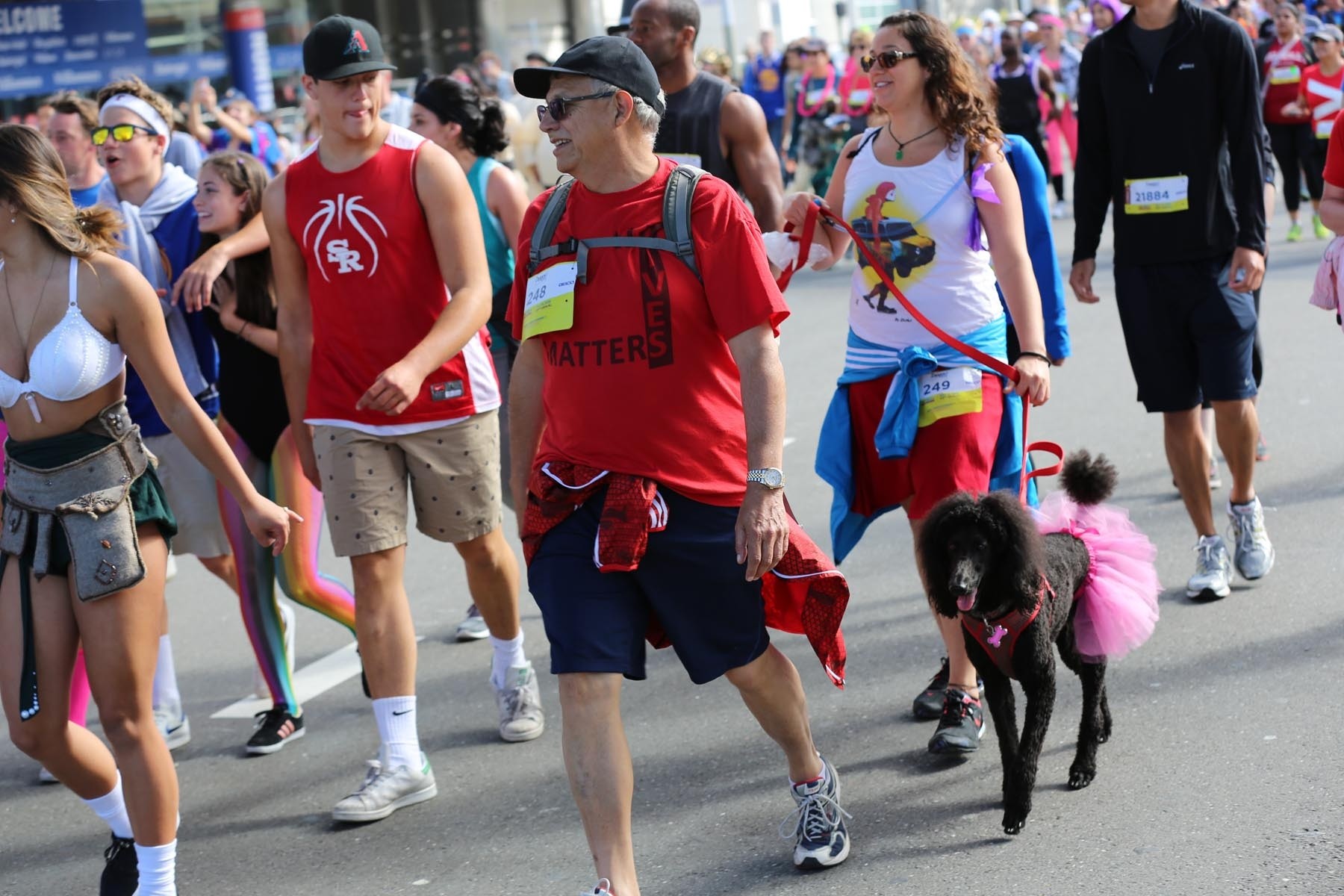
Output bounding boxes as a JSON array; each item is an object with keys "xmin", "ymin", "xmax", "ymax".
[{"xmin": 1171, "ymin": 459, "xmax": 1222, "ymax": 488}]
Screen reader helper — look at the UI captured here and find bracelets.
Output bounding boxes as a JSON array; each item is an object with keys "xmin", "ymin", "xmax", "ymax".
[
  {"xmin": 234, "ymin": 318, "xmax": 250, "ymax": 344},
  {"xmin": 1016, "ymin": 351, "xmax": 1053, "ymax": 369}
]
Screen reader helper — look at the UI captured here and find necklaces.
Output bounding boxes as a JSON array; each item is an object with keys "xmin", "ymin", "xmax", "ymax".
[
  {"xmin": 887, "ymin": 120, "xmax": 944, "ymax": 160},
  {"xmin": 1, "ymin": 249, "xmax": 59, "ymax": 425}
]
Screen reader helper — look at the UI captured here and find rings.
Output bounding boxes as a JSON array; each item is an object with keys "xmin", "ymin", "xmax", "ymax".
[{"xmin": 283, "ymin": 506, "xmax": 289, "ymax": 512}]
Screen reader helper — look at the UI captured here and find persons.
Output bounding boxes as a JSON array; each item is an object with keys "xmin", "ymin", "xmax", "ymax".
[
  {"xmin": 501, "ymin": 32, "xmax": 853, "ymax": 895},
  {"xmin": 781, "ymin": 10, "xmax": 1071, "ymax": 753},
  {"xmin": 696, "ymin": 0, "xmax": 1344, "ymax": 243},
  {"xmin": 1064, "ymin": 0, "xmax": 1279, "ymax": 605},
  {"xmin": 260, "ymin": 15, "xmax": 547, "ymax": 824},
  {"xmin": 380, "ymin": 49, "xmax": 574, "ymax": 189},
  {"xmin": 404, "ymin": 75, "xmax": 537, "ymax": 643},
  {"xmin": 626, "ymin": 0, "xmax": 787, "ymax": 296},
  {"xmin": 0, "ymin": 122, "xmax": 307, "ymax": 896},
  {"xmin": 47, "ymin": 69, "xmax": 321, "ymax": 208},
  {"xmin": 85, "ymin": 75, "xmax": 297, "ymax": 755},
  {"xmin": 182, "ymin": 147, "xmax": 374, "ymax": 755}
]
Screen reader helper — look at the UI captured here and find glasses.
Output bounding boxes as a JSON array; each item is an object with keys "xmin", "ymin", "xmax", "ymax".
[
  {"xmin": 785, "ymin": 23, "xmax": 1056, "ymax": 59},
  {"xmin": 92, "ymin": 124, "xmax": 157, "ymax": 147},
  {"xmin": 1311, "ymin": 38, "xmax": 1338, "ymax": 45},
  {"xmin": 535, "ymin": 89, "xmax": 631, "ymax": 122}
]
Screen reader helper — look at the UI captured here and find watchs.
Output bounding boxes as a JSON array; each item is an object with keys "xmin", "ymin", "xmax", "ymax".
[{"xmin": 745, "ymin": 466, "xmax": 786, "ymax": 490}]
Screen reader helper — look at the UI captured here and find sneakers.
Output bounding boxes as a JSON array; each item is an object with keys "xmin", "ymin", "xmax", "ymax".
[
  {"xmin": 244, "ymin": 706, "xmax": 309, "ymax": 754},
  {"xmin": 331, "ymin": 751, "xmax": 438, "ymax": 822},
  {"xmin": 1254, "ymin": 431, "xmax": 1269, "ymax": 462},
  {"xmin": 1228, "ymin": 495, "xmax": 1274, "ymax": 582},
  {"xmin": 455, "ymin": 603, "xmax": 489, "ymax": 641},
  {"xmin": 911, "ymin": 657, "xmax": 985, "ymax": 717},
  {"xmin": 492, "ymin": 653, "xmax": 545, "ymax": 741},
  {"xmin": 153, "ymin": 712, "xmax": 191, "ymax": 751},
  {"xmin": 1312, "ymin": 212, "xmax": 1329, "ymax": 238},
  {"xmin": 1186, "ymin": 535, "xmax": 1229, "ymax": 600},
  {"xmin": 787, "ymin": 753, "xmax": 852, "ymax": 869},
  {"xmin": 1286, "ymin": 224, "xmax": 1303, "ymax": 242},
  {"xmin": 98, "ymin": 809, "xmax": 181, "ymax": 896},
  {"xmin": 253, "ymin": 604, "xmax": 299, "ymax": 699},
  {"xmin": 931, "ymin": 688, "xmax": 986, "ymax": 755}
]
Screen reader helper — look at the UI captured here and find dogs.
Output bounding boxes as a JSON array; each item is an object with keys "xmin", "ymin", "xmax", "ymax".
[{"xmin": 917, "ymin": 449, "xmax": 1168, "ymax": 836}]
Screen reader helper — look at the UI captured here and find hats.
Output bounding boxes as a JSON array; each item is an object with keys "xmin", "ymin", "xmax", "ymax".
[
  {"xmin": 1309, "ymin": 24, "xmax": 1342, "ymax": 41},
  {"xmin": 447, "ymin": 0, "xmax": 1121, "ymax": 94},
  {"xmin": 222, "ymin": 87, "xmax": 247, "ymax": 110},
  {"xmin": 513, "ymin": 34, "xmax": 666, "ymax": 118},
  {"xmin": 301, "ymin": 13, "xmax": 398, "ymax": 81}
]
